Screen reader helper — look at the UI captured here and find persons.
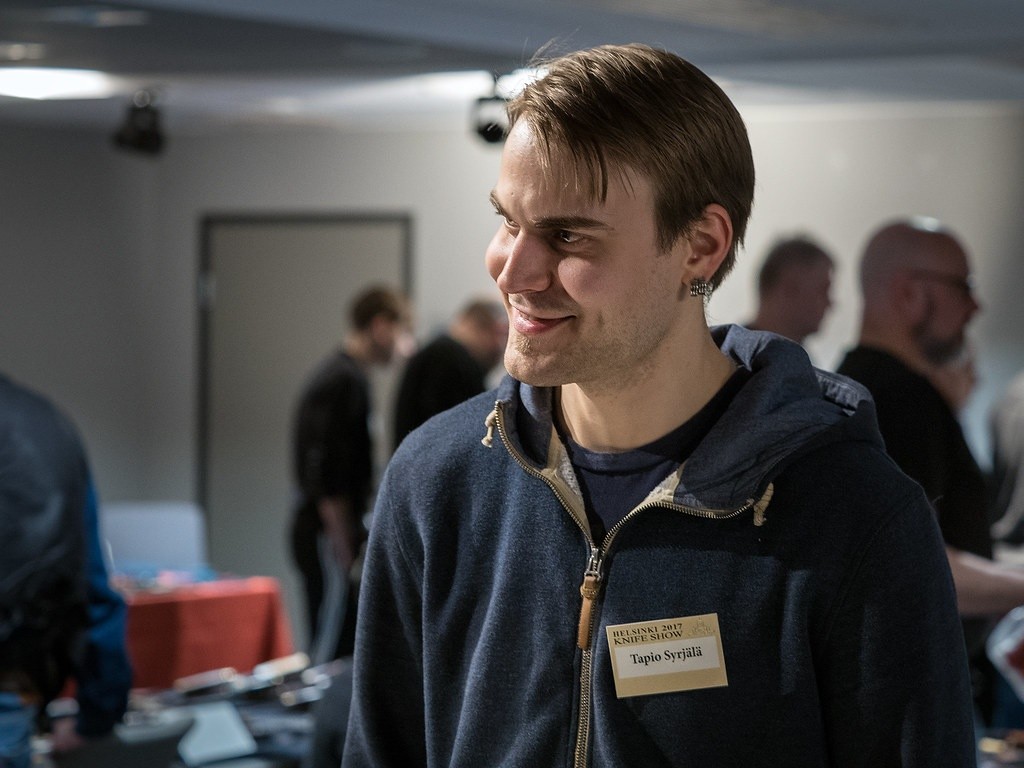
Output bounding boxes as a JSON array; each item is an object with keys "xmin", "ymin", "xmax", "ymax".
[
  {"xmin": 287, "ymin": 218, "xmax": 1024, "ymax": 768},
  {"xmin": 0, "ymin": 373, "xmax": 133, "ymax": 768},
  {"xmin": 340, "ymin": 41, "xmax": 978, "ymax": 768}
]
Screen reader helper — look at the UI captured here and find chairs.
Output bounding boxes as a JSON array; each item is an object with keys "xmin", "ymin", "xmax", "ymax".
[{"xmin": 97, "ymin": 500, "xmax": 208, "ymax": 574}]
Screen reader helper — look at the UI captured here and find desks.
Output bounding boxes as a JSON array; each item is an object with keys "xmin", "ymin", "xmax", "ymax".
[{"xmin": 63, "ymin": 575, "xmax": 296, "ymax": 698}]
